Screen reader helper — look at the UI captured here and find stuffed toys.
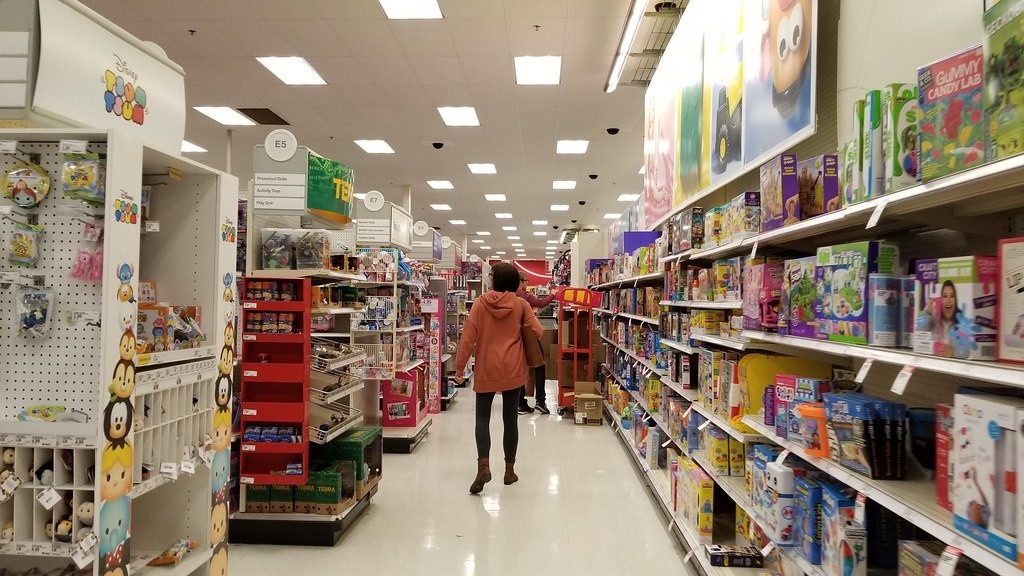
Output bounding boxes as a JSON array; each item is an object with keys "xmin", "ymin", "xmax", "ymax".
[
  {"xmin": 192, "ymin": 397, "xmax": 200, "ymax": 413},
  {"xmin": 134, "ymin": 415, "xmax": 143, "ymax": 431},
  {"xmin": 191, "ymin": 433, "xmax": 214, "ymax": 457},
  {"xmin": 0, "ymin": 447, "xmax": 95, "ymax": 541}
]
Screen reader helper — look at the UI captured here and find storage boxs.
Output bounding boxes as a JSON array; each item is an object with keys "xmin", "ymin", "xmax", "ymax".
[
  {"xmin": 522, "ymin": 0, "xmax": 1024, "ymax": 576},
  {"xmin": 382, "ymin": 365, "xmax": 429, "ymax": 427},
  {"xmin": 244, "ymin": 427, "xmax": 383, "ymax": 515}
]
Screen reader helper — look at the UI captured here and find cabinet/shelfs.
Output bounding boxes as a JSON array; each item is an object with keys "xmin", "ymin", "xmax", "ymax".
[
  {"xmin": 366, "ymin": 279, "xmax": 433, "ymax": 453},
  {"xmin": 741, "ymin": 151, "xmax": 1023, "ymax": 576},
  {"xmin": 423, "ymin": 276, "xmax": 459, "ymax": 410},
  {"xmin": 228, "ymin": 266, "xmax": 378, "ymax": 546},
  {"xmin": 684, "ymin": 236, "xmax": 751, "ymax": 576},
  {"xmin": 590, "ymin": 272, "xmax": 664, "ymax": 472},
  {"xmin": 644, "ymin": 248, "xmax": 700, "ymax": 518},
  {"xmin": 462, "ymin": 261, "xmax": 491, "ymax": 367},
  {"xmin": 446, "ymin": 292, "xmax": 473, "ymax": 387}
]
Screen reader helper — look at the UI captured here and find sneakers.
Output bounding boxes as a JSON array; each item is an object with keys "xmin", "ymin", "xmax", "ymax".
[
  {"xmin": 517, "ymin": 400, "xmax": 535, "ymax": 414},
  {"xmin": 535, "ymin": 399, "xmax": 550, "ymax": 414}
]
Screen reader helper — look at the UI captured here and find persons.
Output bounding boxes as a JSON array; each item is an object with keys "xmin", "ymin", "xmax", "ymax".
[
  {"xmin": 748, "ymin": 268, "xmax": 762, "ymax": 304},
  {"xmin": 784, "ymin": 199, "xmax": 798, "ymax": 225},
  {"xmin": 515, "ymin": 272, "xmax": 556, "ymax": 414},
  {"xmin": 806, "ymin": 433, "xmax": 821, "ymax": 449},
  {"xmin": 415, "ymin": 299, "xmax": 421, "ymax": 311},
  {"xmin": 915, "ymin": 280, "xmax": 970, "ymax": 358},
  {"xmin": 454, "ymin": 263, "xmax": 544, "ymax": 493},
  {"xmin": 732, "ymin": 276, "xmax": 738, "ymax": 291}
]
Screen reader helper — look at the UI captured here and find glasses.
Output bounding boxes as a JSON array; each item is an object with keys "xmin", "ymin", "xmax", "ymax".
[
  {"xmin": 521, "ymin": 279, "xmax": 528, "ymax": 283},
  {"xmin": 488, "ymin": 272, "xmax": 494, "ymax": 277}
]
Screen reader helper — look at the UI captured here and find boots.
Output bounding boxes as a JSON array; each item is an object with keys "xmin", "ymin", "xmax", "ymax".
[
  {"xmin": 504, "ymin": 460, "xmax": 518, "ymax": 485},
  {"xmin": 469, "ymin": 457, "xmax": 491, "ymax": 493}
]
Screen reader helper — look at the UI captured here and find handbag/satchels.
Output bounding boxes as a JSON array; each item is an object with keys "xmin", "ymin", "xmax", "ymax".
[{"xmin": 519, "ymin": 297, "xmax": 546, "ymax": 367}]
